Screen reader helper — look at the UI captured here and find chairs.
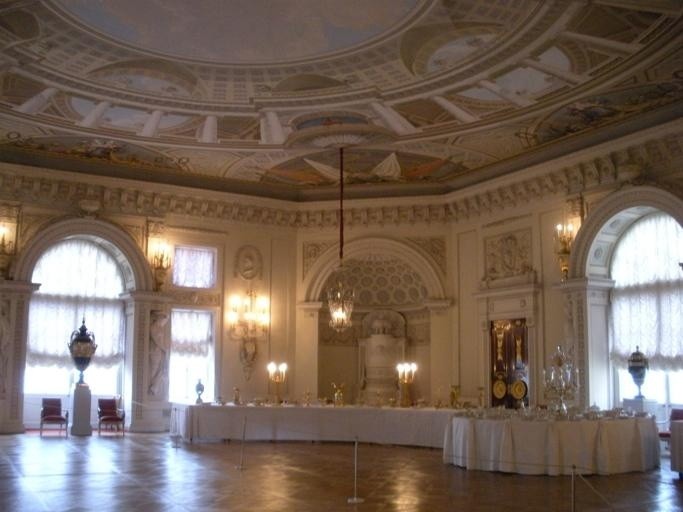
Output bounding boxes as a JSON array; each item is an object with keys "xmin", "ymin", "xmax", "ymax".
[
  {"xmin": 40, "ymin": 397, "xmax": 69, "ymax": 439},
  {"xmin": 96, "ymin": 398, "xmax": 125, "ymax": 436}
]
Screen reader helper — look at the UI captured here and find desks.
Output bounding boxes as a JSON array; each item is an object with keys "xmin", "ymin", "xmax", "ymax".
[
  {"xmin": 169, "ymin": 401, "xmax": 469, "ymax": 450},
  {"xmin": 442, "ymin": 409, "xmax": 662, "ymax": 476}
]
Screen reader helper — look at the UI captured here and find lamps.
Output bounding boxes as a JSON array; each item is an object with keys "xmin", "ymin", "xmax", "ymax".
[
  {"xmin": 557, "ymin": 223, "xmax": 573, "ymax": 254},
  {"xmin": 226, "ymin": 294, "xmax": 269, "ymax": 382},
  {"xmin": 326, "ymin": 144, "xmax": 355, "ymax": 334},
  {"xmin": 153, "ymin": 250, "xmax": 172, "ymax": 286},
  {"xmin": 266, "ymin": 360, "xmax": 287, "ymax": 406},
  {"xmin": 396, "ymin": 362, "xmax": 416, "ymax": 407},
  {"xmin": 540, "ymin": 345, "xmax": 579, "ymax": 418}
]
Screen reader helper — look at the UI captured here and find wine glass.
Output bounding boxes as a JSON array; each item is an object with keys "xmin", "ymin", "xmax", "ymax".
[
  {"xmin": 473, "ymin": 402, "xmax": 647, "ymax": 420},
  {"xmin": 205, "ymin": 393, "xmax": 466, "ymax": 410}
]
